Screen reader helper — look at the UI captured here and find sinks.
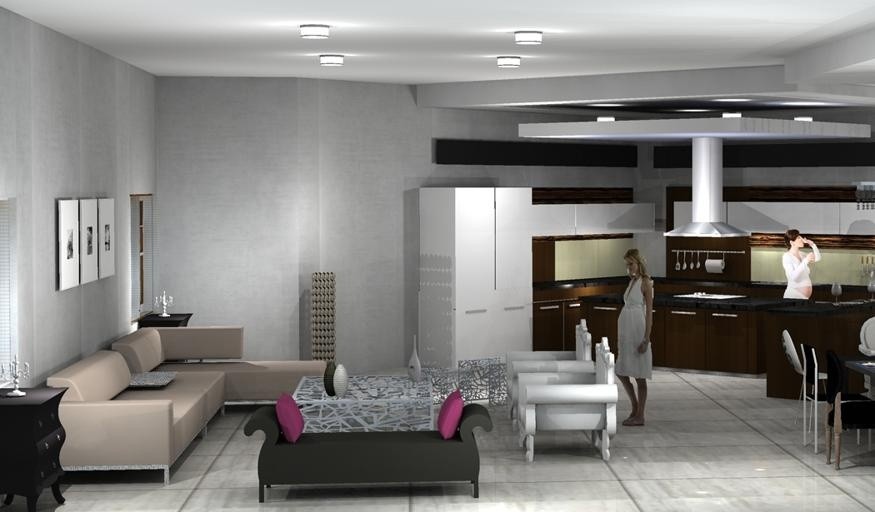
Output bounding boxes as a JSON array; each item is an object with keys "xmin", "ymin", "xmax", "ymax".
[{"xmin": 675, "ymin": 293, "xmax": 746, "ymax": 300}]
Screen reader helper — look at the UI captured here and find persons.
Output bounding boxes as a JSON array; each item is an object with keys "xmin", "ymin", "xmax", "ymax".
[
  {"xmin": 781, "ymin": 228, "xmax": 821, "ymax": 301},
  {"xmin": 614, "ymin": 248, "xmax": 654, "ymax": 427}
]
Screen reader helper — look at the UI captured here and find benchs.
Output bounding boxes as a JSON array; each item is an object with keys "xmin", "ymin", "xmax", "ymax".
[{"xmin": 245, "ymin": 399, "xmax": 493, "ymax": 503}]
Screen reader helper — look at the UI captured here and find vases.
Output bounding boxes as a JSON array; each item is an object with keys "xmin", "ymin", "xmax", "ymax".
[{"xmin": 323, "ymin": 359, "xmax": 349, "ymax": 398}]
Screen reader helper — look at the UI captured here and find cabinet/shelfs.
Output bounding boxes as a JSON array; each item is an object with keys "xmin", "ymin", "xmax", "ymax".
[
  {"xmin": 1, "ymin": 385, "xmax": 70, "ymax": 512},
  {"xmin": 532, "ymin": 294, "xmax": 758, "ymax": 375},
  {"xmin": 138, "ymin": 314, "xmax": 192, "ymax": 326}
]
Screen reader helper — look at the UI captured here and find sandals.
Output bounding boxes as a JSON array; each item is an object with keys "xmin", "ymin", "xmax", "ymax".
[{"xmin": 623, "ymin": 414, "xmax": 645, "ymax": 426}]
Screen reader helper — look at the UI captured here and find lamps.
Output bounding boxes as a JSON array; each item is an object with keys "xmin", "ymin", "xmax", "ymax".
[{"xmin": 299, "ymin": 25, "xmax": 543, "ymax": 70}]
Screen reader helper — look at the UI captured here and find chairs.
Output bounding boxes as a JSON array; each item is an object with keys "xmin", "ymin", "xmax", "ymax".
[
  {"xmin": 504, "ymin": 317, "xmax": 619, "ymax": 463},
  {"xmin": 781, "ymin": 316, "xmax": 874, "ymax": 471}
]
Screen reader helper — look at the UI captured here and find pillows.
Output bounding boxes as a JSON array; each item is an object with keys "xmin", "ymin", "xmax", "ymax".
[
  {"xmin": 437, "ymin": 389, "xmax": 465, "ymax": 441},
  {"xmin": 276, "ymin": 391, "xmax": 304, "ymax": 444}
]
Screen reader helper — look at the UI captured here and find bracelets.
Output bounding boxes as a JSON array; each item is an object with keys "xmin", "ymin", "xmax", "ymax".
[{"xmin": 642, "ymin": 339, "xmax": 649, "ymax": 344}]
